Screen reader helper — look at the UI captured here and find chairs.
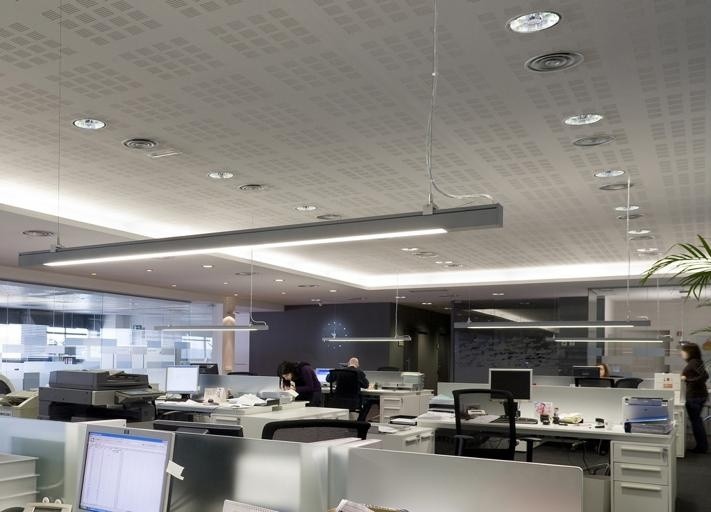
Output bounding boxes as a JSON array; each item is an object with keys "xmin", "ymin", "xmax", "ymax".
[
  {"xmin": 573, "ymin": 364, "xmax": 600, "ymax": 388},
  {"xmin": 452, "ymin": 388, "xmax": 516, "ymax": 460},
  {"xmin": 617, "ymin": 377, "xmax": 643, "ymax": 388},
  {"xmin": 261, "ymin": 419, "xmax": 372, "ymax": 441},
  {"xmin": 575, "ymin": 376, "xmax": 615, "ymax": 387}
]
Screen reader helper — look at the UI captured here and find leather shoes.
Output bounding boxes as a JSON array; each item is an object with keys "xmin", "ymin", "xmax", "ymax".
[{"xmin": 689, "ymin": 446, "xmax": 708, "ymax": 454}]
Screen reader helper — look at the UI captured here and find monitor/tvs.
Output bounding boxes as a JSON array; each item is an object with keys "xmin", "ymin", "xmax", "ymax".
[
  {"xmin": 573, "ymin": 367, "xmax": 601, "ymax": 379},
  {"xmin": 489, "ymin": 369, "xmax": 532, "ymax": 401},
  {"xmin": 77, "ymin": 426, "xmax": 175, "ymax": 512},
  {"xmin": 165, "ymin": 366, "xmax": 199, "ymax": 402}
]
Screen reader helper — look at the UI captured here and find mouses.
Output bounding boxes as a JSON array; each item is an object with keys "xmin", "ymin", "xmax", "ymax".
[{"xmin": 543, "ymin": 419, "xmax": 550, "ymax": 425}]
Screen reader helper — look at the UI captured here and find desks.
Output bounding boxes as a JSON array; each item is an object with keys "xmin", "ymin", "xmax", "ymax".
[
  {"xmin": 321, "ymin": 384, "xmax": 434, "ymax": 427},
  {"xmin": 155, "ymin": 392, "xmax": 349, "ymax": 439},
  {"xmin": 416, "ymin": 408, "xmax": 677, "ymax": 512},
  {"xmin": 673, "ymin": 394, "xmax": 687, "ymax": 459}
]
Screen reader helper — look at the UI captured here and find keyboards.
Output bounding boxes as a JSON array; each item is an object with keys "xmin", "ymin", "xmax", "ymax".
[{"xmin": 491, "ymin": 417, "xmax": 538, "ymax": 424}]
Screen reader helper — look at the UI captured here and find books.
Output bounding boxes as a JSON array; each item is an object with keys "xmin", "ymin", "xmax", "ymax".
[
  {"xmin": 389, "ymin": 418, "xmax": 417, "ymax": 427},
  {"xmin": 227, "ymin": 393, "xmax": 267, "ymax": 407},
  {"xmin": 624, "ymin": 416, "xmax": 673, "ymax": 435},
  {"xmin": 279, "ymin": 391, "xmax": 295, "ymax": 405}
]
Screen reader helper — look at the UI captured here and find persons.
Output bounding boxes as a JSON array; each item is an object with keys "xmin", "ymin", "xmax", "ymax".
[
  {"xmin": 325, "ymin": 358, "xmax": 370, "ymax": 416},
  {"xmin": 277, "ymin": 360, "xmax": 322, "ymax": 407},
  {"xmin": 597, "ymin": 363, "xmax": 608, "ymax": 377},
  {"xmin": 681, "ymin": 342, "xmax": 710, "ymax": 455}
]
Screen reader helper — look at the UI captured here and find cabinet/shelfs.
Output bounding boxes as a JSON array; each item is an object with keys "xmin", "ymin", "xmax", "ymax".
[{"xmin": 365, "ymin": 423, "xmax": 436, "ymax": 453}]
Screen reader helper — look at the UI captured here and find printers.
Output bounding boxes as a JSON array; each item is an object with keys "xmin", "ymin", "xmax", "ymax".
[
  {"xmin": 38, "ymin": 370, "xmax": 164, "ymax": 421},
  {"xmin": 0, "ymin": 391, "xmax": 37, "ymax": 420}
]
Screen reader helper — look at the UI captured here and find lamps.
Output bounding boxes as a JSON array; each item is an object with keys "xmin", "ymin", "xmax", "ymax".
[
  {"xmin": 322, "ymin": 290, "xmax": 411, "ymax": 343},
  {"xmin": 154, "ymin": 251, "xmax": 268, "ymax": 334},
  {"xmin": 17, "ymin": 2, "xmax": 505, "ymax": 268},
  {"xmin": 546, "ymin": 256, "xmax": 673, "ymax": 344},
  {"xmin": 453, "ymin": 174, "xmax": 652, "ymax": 329},
  {"xmin": 223, "ymin": 310, "xmax": 236, "ymax": 323}
]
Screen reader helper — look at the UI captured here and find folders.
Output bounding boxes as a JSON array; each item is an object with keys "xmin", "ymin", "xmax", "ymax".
[
  {"xmin": 390, "ymin": 414, "xmax": 417, "ymax": 426},
  {"xmin": 624, "ymin": 415, "xmax": 669, "ymax": 433}
]
